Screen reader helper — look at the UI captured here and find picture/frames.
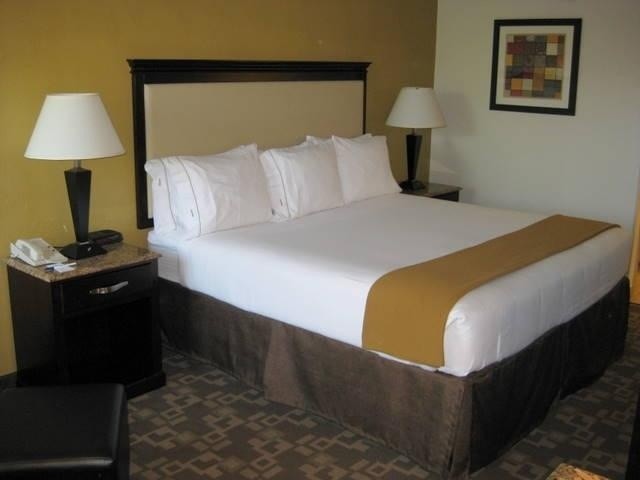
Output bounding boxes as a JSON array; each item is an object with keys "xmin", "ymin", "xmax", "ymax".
[{"xmin": 488, "ymin": 18, "xmax": 582, "ymax": 117}]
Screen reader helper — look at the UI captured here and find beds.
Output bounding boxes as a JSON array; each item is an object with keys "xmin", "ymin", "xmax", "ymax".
[{"xmin": 125, "ymin": 58, "xmax": 630, "ymax": 479}]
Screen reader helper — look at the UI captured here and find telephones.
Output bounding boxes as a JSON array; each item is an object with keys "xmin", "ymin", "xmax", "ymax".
[{"xmin": 10, "ymin": 237, "xmax": 68, "ymax": 267}]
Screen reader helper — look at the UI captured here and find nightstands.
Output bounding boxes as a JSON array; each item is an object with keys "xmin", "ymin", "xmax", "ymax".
[
  {"xmin": 7, "ymin": 241, "xmax": 166, "ymax": 400},
  {"xmin": 399, "ymin": 181, "xmax": 464, "ymax": 202}
]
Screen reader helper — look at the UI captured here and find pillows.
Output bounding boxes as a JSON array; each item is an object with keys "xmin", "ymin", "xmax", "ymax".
[
  {"xmin": 259, "ymin": 140, "xmax": 344, "ymax": 222},
  {"xmin": 144, "ymin": 143, "xmax": 278, "ymax": 246},
  {"xmin": 306, "ymin": 133, "xmax": 373, "ymax": 141},
  {"xmin": 332, "ymin": 136, "xmax": 402, "ymax": 205}
]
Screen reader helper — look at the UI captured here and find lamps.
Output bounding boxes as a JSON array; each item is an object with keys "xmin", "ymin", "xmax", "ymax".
[
  {"xmin": 24, "ymin": 92, "xmax": 124, "ymax": 261},
  {"xmin": 385, "ymin": 87, "xmax": 448, "ymax": 193}
]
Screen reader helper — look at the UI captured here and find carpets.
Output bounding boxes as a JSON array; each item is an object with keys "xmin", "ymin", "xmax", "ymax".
[{"xmin": 126, "ymin": 302, "xmax": 640, "ymax": 480}]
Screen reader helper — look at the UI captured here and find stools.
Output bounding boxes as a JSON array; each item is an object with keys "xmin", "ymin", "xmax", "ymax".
[{"xmin": 0, "ymin": 384, "xmax": 130, "ymax": 480}]
